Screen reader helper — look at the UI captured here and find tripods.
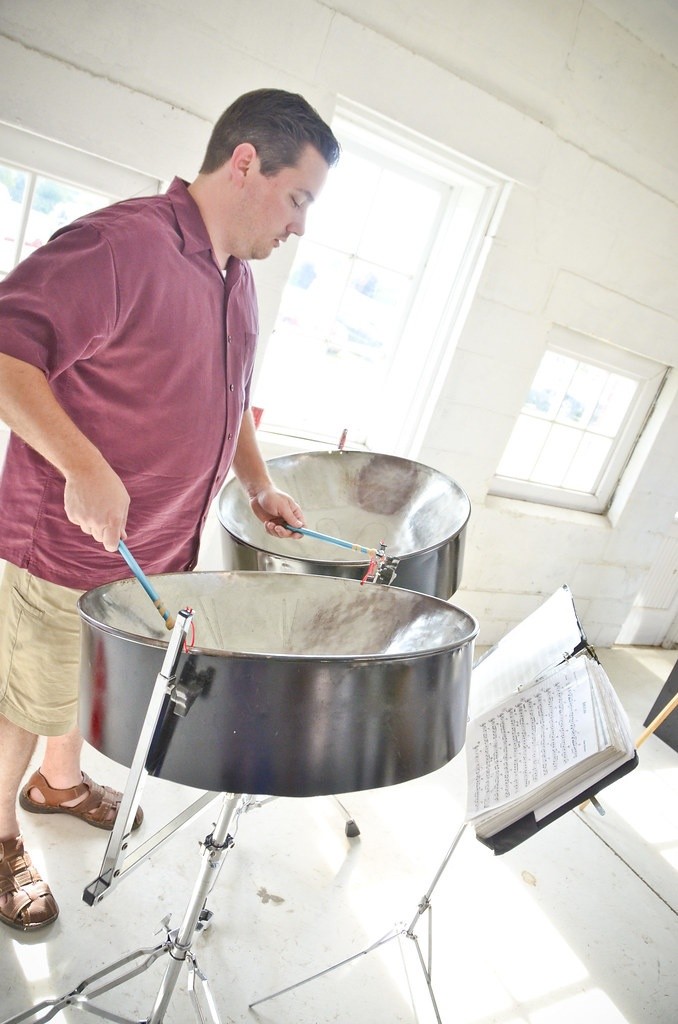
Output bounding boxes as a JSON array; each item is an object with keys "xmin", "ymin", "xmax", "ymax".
[
  {"xmin": 0, "ymin": 792, "xmax": 240, "ymax": 1024},
  {"xmin": 249, "ymin": 585, "xmax": 639, "ymax": 1024}
]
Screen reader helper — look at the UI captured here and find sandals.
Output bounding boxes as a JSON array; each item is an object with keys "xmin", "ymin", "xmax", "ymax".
[
  {"xmin": 19, "ymin": 766, "xmax": 144, "ymax": 832},
  {"xmin": 0, "ymin": 835, "xmax": 59, "ymax": 931}
]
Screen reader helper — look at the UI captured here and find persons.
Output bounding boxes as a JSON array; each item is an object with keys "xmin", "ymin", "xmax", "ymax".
[{"xmin": 1, "ymin": 88, "xmax": 340, "ymax": 932}]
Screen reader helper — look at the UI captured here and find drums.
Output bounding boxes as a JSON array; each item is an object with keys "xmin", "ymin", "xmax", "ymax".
[
  {"xmin": 211, "ymin": 447, "xmax": 476, "ymax": 607},
  {"xmin": 74, "ymin": 565, "xmax": 478, "ymax": 801}
]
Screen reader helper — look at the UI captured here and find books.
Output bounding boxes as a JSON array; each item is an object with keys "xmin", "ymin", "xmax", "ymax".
[{"xmin": 464, "ymin": 585, "xmax": 638, "ymax": 856}]
